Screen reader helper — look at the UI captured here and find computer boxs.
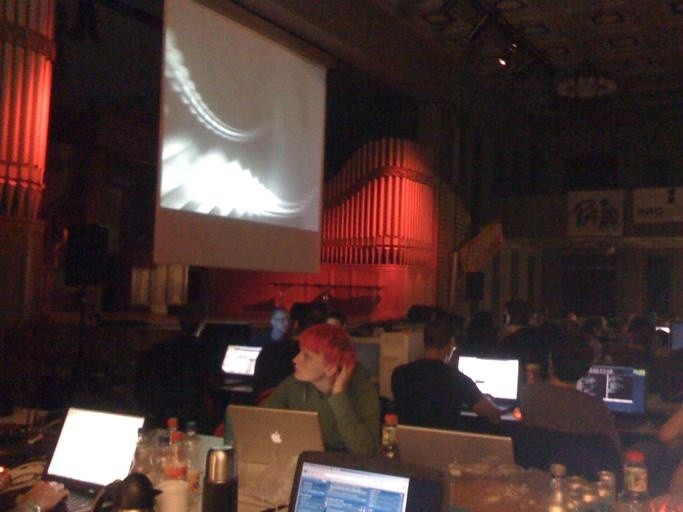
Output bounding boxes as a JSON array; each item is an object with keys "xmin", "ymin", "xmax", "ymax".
[{"xmin": 379, "ymin": 328, "xmax": 424, "ymax": 401}]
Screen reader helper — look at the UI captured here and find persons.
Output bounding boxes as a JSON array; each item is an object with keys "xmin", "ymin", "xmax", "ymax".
[{"xmin": 0, "ymin": 299, "xmax": 682, "ymax": 512}]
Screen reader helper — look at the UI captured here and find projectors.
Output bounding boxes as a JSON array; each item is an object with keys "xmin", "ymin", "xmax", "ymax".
[{"xmin": 462, "ymin": 14, "xmax": 535, "ymax": 82}]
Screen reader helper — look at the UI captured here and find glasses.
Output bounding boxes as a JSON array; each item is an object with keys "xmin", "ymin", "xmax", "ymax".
[{"xmin": 281, "ymin": 318, "xmax": 293, "ymax": 323}]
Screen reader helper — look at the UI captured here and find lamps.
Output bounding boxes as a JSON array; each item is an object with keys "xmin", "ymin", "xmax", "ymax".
[{"xmin": 497, "ymin": 40, "xmax": 519, "ymax": 66}]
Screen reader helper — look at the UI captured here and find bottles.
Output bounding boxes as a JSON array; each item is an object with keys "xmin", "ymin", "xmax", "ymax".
[
  {"xmin": 378, "ymin": 413, "xmax": 400, "ymax": 460},
  {"xmin": 547, "ymin": 450, "xmax": 651, "ymax": 512},
  {"xmin": 133, "ymin": 417, "xmax": 203, "ymax": 504}
]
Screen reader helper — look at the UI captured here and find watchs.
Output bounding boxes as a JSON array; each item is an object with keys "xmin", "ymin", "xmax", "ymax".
[{"xmin": 23, "ymin": 501, "xmax": 40, "ymax": 510}]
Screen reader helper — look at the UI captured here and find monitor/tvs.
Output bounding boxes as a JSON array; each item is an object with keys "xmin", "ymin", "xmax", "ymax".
[
  {"xmin": 669, "ymin": 320, "xmax": 683, "ymax": 358},
  {"xmin": 346, "ymin": 336, "xmax": 381, "ymax": 380}
]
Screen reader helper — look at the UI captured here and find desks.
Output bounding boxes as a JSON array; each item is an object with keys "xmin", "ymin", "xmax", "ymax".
[{"xmin": 0, "ymin": 311, "xmax": 681, "ymax": 512}]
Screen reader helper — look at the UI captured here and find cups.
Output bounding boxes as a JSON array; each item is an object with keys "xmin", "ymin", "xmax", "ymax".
[
  {"xmin": 527, "ymin": 364, "xmax": 539, "ymax": 383},
  {"xmin": 203, "ymin": 444, "xmax": 238, "ymax": 488},
  {"xmin": 152, "ymin": 480, "xmax": 189, "ymax": 512}
]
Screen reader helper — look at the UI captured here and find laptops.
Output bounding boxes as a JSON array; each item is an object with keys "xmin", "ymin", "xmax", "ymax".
[
  {"xmin": 228, "ymin": 405, "xmax": 326, "ymax": 462},
  {"xmin": 456, "ymin": 354, "xmax": 521, "ymax": 413},
  {"xmin": 288, "ymin": 452, "xmax": 412, "ymax": 512},
  {"xmin": 220, "ymin": 343, "xmax": 262, "ymax": 385},
  {"xmin": 40, "ymin": 404, "xmax": 146, "ymax": 512},
  {"xmin": 398, "ymin": 424, "xmax": 513, "ymax": 476},
  {"xmin": 577, "ymin": 364, "xmax": 647, "ymax": 426}
]
